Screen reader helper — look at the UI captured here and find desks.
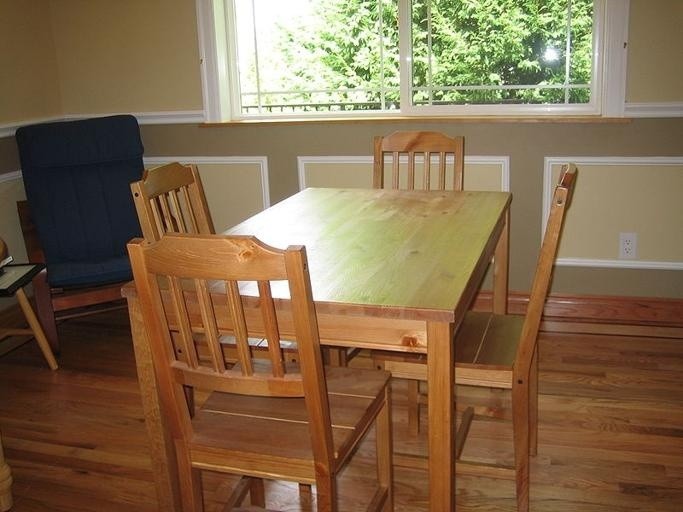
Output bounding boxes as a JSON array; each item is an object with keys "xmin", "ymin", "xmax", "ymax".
[{"xmin": 121, "ymin": 186, "xmax": 513, "ymax": 512}]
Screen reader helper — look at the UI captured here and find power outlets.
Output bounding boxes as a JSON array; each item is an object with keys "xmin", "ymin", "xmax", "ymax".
[{"xmin": 617, "ymin": 231, "xmax": 637, "ymax": 260}]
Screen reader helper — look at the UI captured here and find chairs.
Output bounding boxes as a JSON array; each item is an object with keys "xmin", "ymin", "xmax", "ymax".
[
  {"xmin": 14, "ymin": 113, "xmax": 178, "ymax": 357},
  {"xmin": 368, "ymin": 163, "xmax": 578, "ymax": 510},
  {"xmin": 125, "ymin": 234, "xmax": 395, "ymax": 512},
  {"xmin": 130, "ymin": 163, "xmax": 357, "ymax": 367},
  {"xmin": 373, "ymin": 129, "xmax": 466, "ymax": 191}
]
